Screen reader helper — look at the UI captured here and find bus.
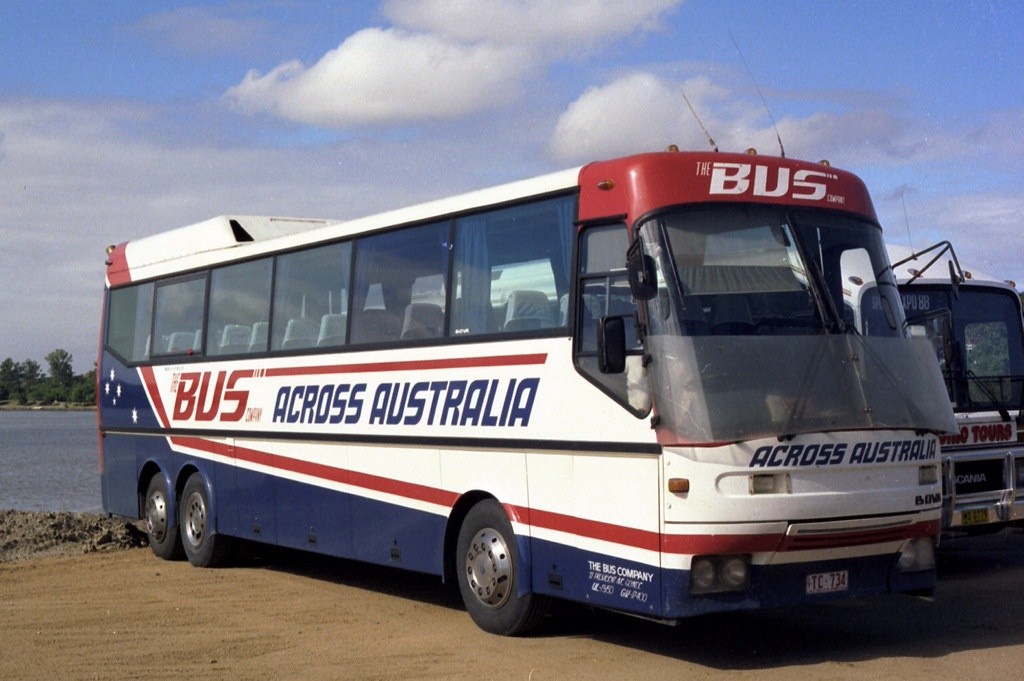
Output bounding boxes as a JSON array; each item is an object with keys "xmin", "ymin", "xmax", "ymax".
[
  {"xmin": 825, "ymin": 241, "xmax": 1023, "ymax": 553},
  {"xmin": 95, "ymin": 145, "xmax": 962, "ymax": 637}
]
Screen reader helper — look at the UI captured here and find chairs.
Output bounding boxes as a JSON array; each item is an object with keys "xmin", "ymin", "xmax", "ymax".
[{"xmin": 142, "ymin": 291, "xmax": 629, "ymax": 402}]
